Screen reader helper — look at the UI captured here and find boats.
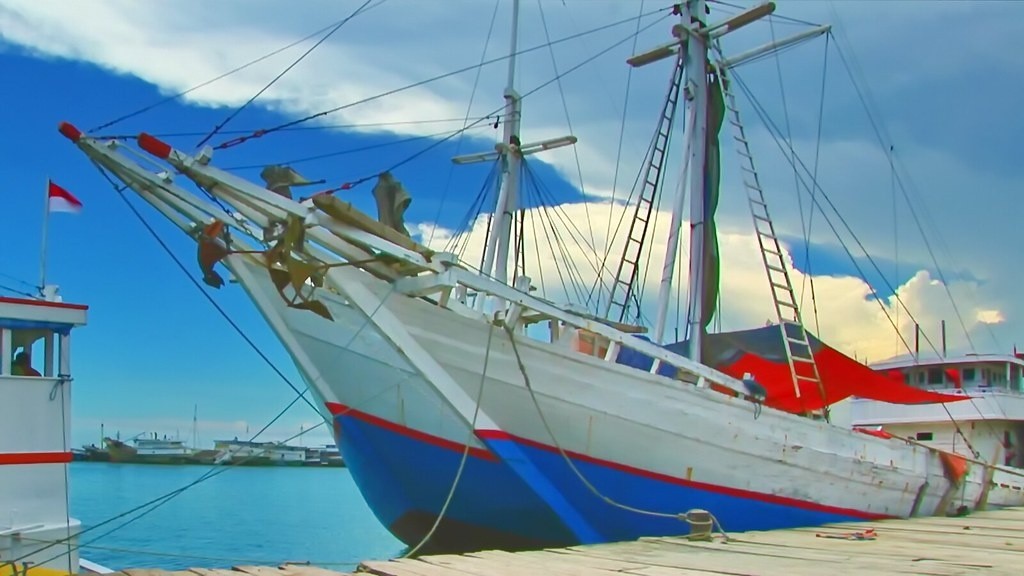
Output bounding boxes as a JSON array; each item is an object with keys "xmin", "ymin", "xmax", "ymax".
[
  {"xmin": 79, "ymin": 436, "xmax": 222, "ymax": 465},
  {"xmin": 59, "ymin": 0, "xmax": 1024, "ymax": 557}
]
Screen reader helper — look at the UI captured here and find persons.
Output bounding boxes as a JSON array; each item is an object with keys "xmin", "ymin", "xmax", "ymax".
[{"xmin": 11, "ymin": 352, "xmax": 41, "ymax": 377}]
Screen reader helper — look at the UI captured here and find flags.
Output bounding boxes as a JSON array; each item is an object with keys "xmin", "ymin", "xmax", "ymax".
[{"xmin": 48, "ymin": 183, "xmax": 82, "ymax": 213}]
